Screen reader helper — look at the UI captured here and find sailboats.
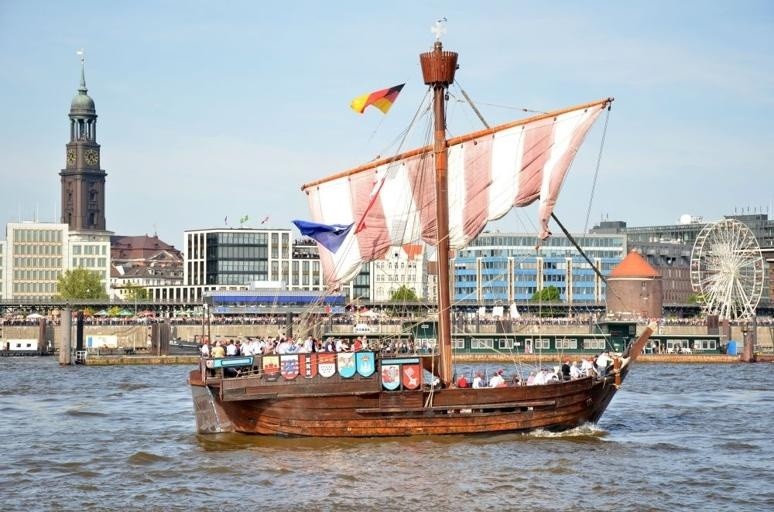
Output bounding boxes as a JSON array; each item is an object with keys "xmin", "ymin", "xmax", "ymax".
[{"xmin": 187, "ymin": 17, "xmax": 653, "ymax": 438}]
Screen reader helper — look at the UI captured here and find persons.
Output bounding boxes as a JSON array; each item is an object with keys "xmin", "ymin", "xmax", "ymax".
[
  {"xmin": 458, "ymin": 349, "xmax": 630, "ymax": 388},
  {"xmin": 1, "ymin": 314, "xmax": 395, "ymax": 325},
  {"xmin": 452, "ymin": 316, "xmax": 774, "ymax": 326},
  {"xmin": 196, "ymin": 333, "xmax": 435, "ymax": 358}
]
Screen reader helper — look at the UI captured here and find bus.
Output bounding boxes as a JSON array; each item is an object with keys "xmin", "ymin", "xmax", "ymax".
[{"xmin": 321, "ymin": 332, "xmax": 414, "ymax": 355}]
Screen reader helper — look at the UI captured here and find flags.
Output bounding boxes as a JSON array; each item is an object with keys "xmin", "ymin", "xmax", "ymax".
[
  {"xmin": 240, "ymin": 214, "xmax": 248, "ymax": 223},
  {"xmin": 350, "ymin": 82, "xmax": 407, "ymax": 114},
  {"xmin": 291, "ymin": 219, "xmax": 356, "ymax": 254}
]
